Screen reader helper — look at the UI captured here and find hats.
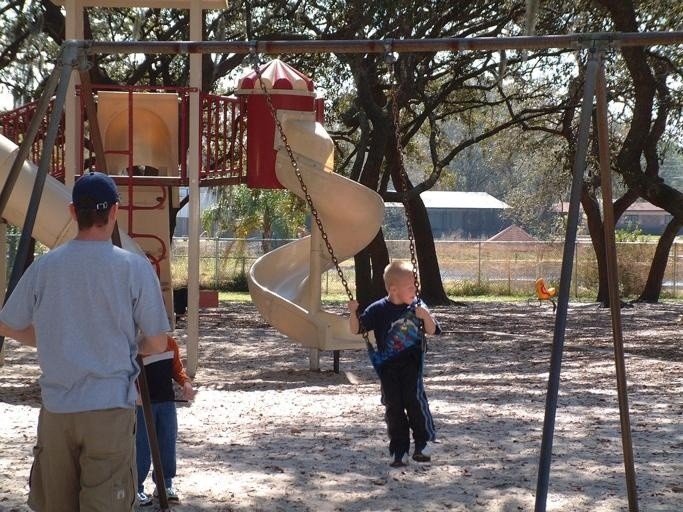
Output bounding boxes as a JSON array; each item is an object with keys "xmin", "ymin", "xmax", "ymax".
[{"xmin": 72, "ymin": 172, "xmax": 120, "ymax": 211}]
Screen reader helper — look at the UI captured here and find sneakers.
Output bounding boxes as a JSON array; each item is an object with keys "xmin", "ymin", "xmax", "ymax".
[
  {"xmin": 152, "ymin": 486, "xmax": 179, "ymax": 504},
  {"xmin": 412, "ymin": 434, "xmax": 433, "ymax": 461},
  {"xmin": 137, "ymin": 492, "xmax": 154, "ymax": 507},
  {"xmin": 389, "ymin": 448, "xmax": 410, "ymax": 466}
]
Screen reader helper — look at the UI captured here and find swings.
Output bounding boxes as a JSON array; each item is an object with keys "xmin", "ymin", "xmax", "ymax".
[{"xmin": 254, "ymin": 52, "xmax": 427, "ymax": 384}]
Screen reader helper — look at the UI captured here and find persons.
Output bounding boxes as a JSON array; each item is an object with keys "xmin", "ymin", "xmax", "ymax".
[
  {"xmin": 124, "ymin": 332, "xmax": 193, "ymax": 507},
  {"xmin": 0, "ymin": 170, "xmax": 168, "ymax": 512},
  {"xmin": 346, "ymin": 258, "xmax": 441, "ymax": 467}
]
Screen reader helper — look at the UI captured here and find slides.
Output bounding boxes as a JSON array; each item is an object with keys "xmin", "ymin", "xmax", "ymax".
[
  {"xmin": 0, "ymin": 132, "xmax": 151, "ymax": 273},
  {"xmin": 247, "ymin": 108, "xmax": 384, "ymax": 351},
  {"xmin": 80, "ymin": 152, "xmax": 189, "ymax": 316}
]
